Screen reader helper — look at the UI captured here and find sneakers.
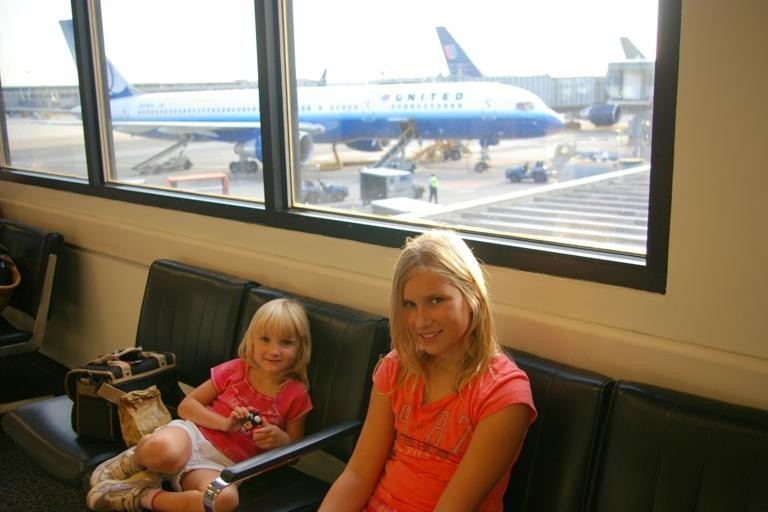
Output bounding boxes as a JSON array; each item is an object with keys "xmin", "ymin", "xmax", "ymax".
[{"xmin": 86, "ymin": 444, "xmax": 164, "ymax": 512}]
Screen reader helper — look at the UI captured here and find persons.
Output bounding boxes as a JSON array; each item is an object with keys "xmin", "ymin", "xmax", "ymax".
[
  {"xmin": 86, "ymin": 299, "xmax": 313, "ymax": 512},
  {"xmin": 316, "ymin": 228, "xmax": 538, "ymax": 512},
  {"xmin": 427, "ymin": 173, "xmax": 439, "ymax": 204}
]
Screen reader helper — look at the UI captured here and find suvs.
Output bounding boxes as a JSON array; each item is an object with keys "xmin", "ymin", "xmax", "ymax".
[{"xmin": 361, "ymin": 167, "xmax": 425, "ymax": 205}]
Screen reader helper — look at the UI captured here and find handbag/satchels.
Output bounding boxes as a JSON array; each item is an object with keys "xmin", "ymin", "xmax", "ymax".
[
  {"xmin": 1, "ymin": 245, "xmax": 22, "ymax": 310},
  {"xmin": 64, "ymin": 346, "xmax": 178, "ymax": 442}
]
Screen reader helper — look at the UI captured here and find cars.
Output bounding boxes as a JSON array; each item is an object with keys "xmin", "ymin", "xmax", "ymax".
[
  {"xmin": 507, "ymin": 161, "xmax": 549, "ymax": 182},
  {"xmin": 301, "ymin": 179, "xmax": 349, "ymax": 204}
]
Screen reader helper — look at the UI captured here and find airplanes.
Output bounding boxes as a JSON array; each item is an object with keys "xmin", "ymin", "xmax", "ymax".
[{"xmin": 60, "ymin": 19, "xmax": 564, "ymax": 178}]
